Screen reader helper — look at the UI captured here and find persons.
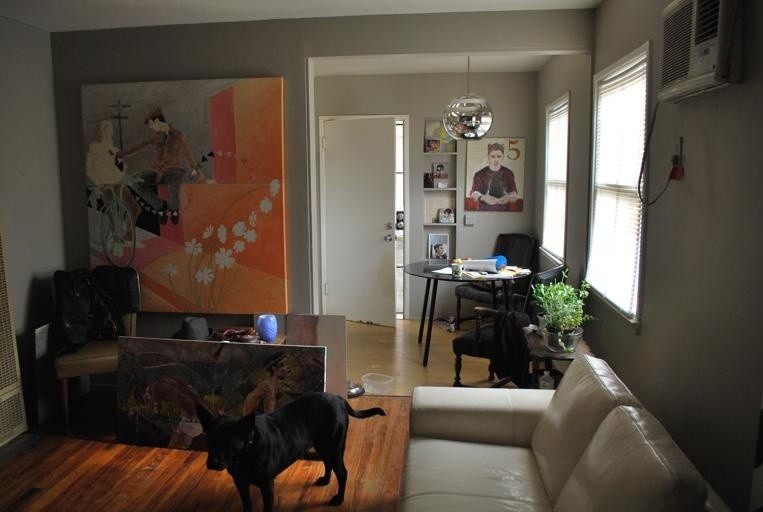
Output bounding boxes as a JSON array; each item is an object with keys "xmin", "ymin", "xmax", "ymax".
[
  {"xmin": 427, "ymin": 139, "xmax": 441, "ymax": 152},
  {"xmin": 434, "ymin": 243, "xmax": 445, "ymax": 260},
  {"xmin": 441, "ymin": 208, "xmax": 455, "ymax": 224},
  {"xmin": 128, "ymin": 378, "xmax": 208, "ymax": 449},
  {"xmin": 469, "ymin": 143, "xmax": 518, "ymax": 211},
  {"xmin": 240, "ymin": 351, "xmax": 294, "ymax": 417},
  {"xmin": 87, "ymin": 105, "xmax": 198, "ymax": 227}
]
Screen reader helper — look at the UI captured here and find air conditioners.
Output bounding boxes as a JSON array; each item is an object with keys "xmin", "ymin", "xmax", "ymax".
[{"xmin": 653, "ymin": 1, "xmax": 746, "ymax": 101}]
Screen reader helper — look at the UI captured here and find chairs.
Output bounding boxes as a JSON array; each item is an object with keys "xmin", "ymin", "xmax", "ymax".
[
  {"xmin": 455, "ymin": 233, "xmax": 539, "ymax": 330},
  {"xmin": 452, "ymin": 264, "xmax": 567, "ymax": 388},
  {"xmin": 51, "ymin": 264, "xmax": 140, "ymax": 436}
]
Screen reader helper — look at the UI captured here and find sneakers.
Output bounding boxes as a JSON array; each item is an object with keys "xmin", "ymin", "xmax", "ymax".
[{"xmin": 157, "ymin": 202, "xmax": 179, "ymax": 224}]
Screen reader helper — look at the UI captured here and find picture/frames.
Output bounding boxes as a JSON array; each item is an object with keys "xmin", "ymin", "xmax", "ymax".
[
  {"xmin": 465, "ymin": 138, "xmax": 526, "ymax": 211},
  {"xmin": 424, "ymin": 120, "xmax": 457, "ymax": 153},
  {"xmin": 80, "ymin": 76, "xmax": 287, "ymax": 318},
  {"xmin": 428, "ymin": 234, "xmax": 449, "ymax": 266},
  {"xmin": 432, "ymin": 243, "xmax": 448, "ymax": 259},
  {"xmin": 438, "ymin": 208, "xmax": 455, "ymax": 224},
  {"xmin": 424, "ymin": 161, "xmax": 449, "ymax": 188},
  {"xmin": 116, "ymin": 334, "xmax": 328, "ymax": 461}
]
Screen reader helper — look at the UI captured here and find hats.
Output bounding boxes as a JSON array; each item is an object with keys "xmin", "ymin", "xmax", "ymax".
[
  {"xmin": 143, "ymin": 108, "xmax": 162, "ymax": 122},
  {"xmin": 173, "ymin": 316, "xmax": 215, "ymax": 340}
]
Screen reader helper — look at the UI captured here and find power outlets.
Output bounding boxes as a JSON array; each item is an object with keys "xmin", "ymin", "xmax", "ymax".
[{"xmin": 671, "ymin": 137, "xmax": 683, "ymax": 180}]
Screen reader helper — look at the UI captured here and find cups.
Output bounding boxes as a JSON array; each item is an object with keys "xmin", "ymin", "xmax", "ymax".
[
  {"xmin": 451, "ymin": 263, "xmax": 465, "ymax": 277},
  {"xmin": 258, "ymin": 315, "xmax": 277, "ymax": 342}
]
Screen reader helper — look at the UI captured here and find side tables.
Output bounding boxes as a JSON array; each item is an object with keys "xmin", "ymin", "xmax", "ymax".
[{"xmin": 522, "ymin": 325, "xmax": 592, "ymax": 387}]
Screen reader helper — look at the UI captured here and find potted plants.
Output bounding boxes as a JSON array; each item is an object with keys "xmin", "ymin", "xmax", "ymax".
[
  {"xmin": 532, "ymin": 268, "xmax": 589, "ymax": 337},
  {"xmin": 533, "ymin": 283, "xmax": 597, "ymax": 355}
]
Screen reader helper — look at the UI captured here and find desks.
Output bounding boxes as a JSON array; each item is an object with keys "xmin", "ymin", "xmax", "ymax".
[
  {"xmin": 403, "ymin": 259, "xmax": 532, "ymax": 367},
  {"xmin": 209, "ymin": 326, "xmax": 286, "ymax": 344}
]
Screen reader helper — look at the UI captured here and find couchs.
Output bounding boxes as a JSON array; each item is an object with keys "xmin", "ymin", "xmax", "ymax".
[{"xmin": 399, "ymin": 357, "xmax": 728, "ymax": 511}]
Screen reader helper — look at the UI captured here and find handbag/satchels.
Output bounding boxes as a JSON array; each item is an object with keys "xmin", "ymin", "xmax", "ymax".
[{"xmin": 54, "ymin": 266, "xmax": 140, "ymax": 339}]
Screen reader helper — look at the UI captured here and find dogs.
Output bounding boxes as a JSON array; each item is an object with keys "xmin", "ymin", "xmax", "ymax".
[{"xmin": 193, "ymin": 391, "xmax": 387, "ymax": 512}]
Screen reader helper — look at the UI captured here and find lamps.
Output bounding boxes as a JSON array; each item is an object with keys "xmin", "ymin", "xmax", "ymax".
[{"xmin": 442, "ymin": 56, "xmax": 493, "ymax": 140}]
